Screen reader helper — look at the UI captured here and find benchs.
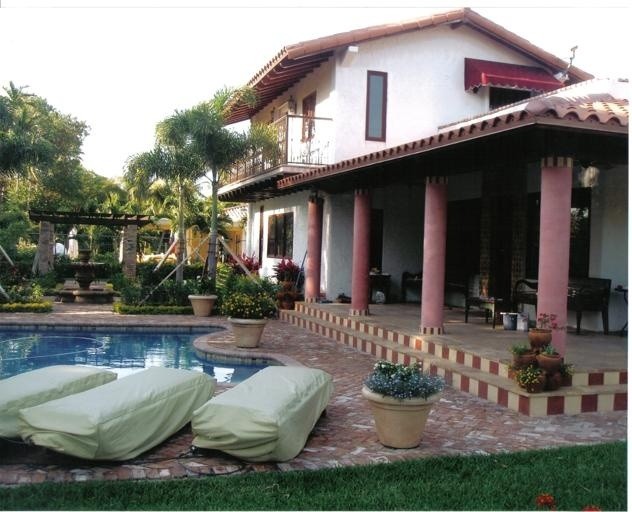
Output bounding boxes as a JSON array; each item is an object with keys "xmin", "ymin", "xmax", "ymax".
[
  {"xmin": 400, "ymin": 268, "xmax": 472, "ymax": 308},
  {"xmin": 512, "ymin": 274, "xmax": 615, "ymax": 335}
]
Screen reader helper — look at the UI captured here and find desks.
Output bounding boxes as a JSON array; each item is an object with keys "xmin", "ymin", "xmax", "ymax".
[
  {"xmin": 613, "ymin": 285, "xmax": 628, "ymax": 337},
  {"xmin": 367, "ymin": 274, "xmax": 392, "ymax": 306},
  {"xmin": 463, "ymin": 297, "xmax": 505, "ymax": 329}
]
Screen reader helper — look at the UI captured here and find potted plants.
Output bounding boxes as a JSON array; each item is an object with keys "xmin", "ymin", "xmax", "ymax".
[{"xmin": 187, "ymin": 274, "xmax": 219, "ymax": 318}]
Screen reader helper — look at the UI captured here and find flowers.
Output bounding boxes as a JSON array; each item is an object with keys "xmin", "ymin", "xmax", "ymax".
[
  {"xmin": 273, "ymin": 259, "xmax": 304, "ymax": 281},
  {"xmin": 223, "ymin": 290, "xmax": 280, "ymax": 319},
  {"xmin": 360, "ymin": 355, "xmax": 446, "ymax": 399}
]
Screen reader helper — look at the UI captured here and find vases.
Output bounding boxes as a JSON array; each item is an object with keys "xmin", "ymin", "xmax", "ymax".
[
  {"xmin": 359, "ymin": 384, "xmax": 442, "ymax": 452},
  {"xmin": 228, "ymin": 315, "xmax": 269, "ymax": 350},
  {"xmin": 277, "ymin": 280, "xmax": 301, "ymax": 308},
  {"xmin": 508, "ymin": 314, "xmax": 571, "ymax": 394}
]
